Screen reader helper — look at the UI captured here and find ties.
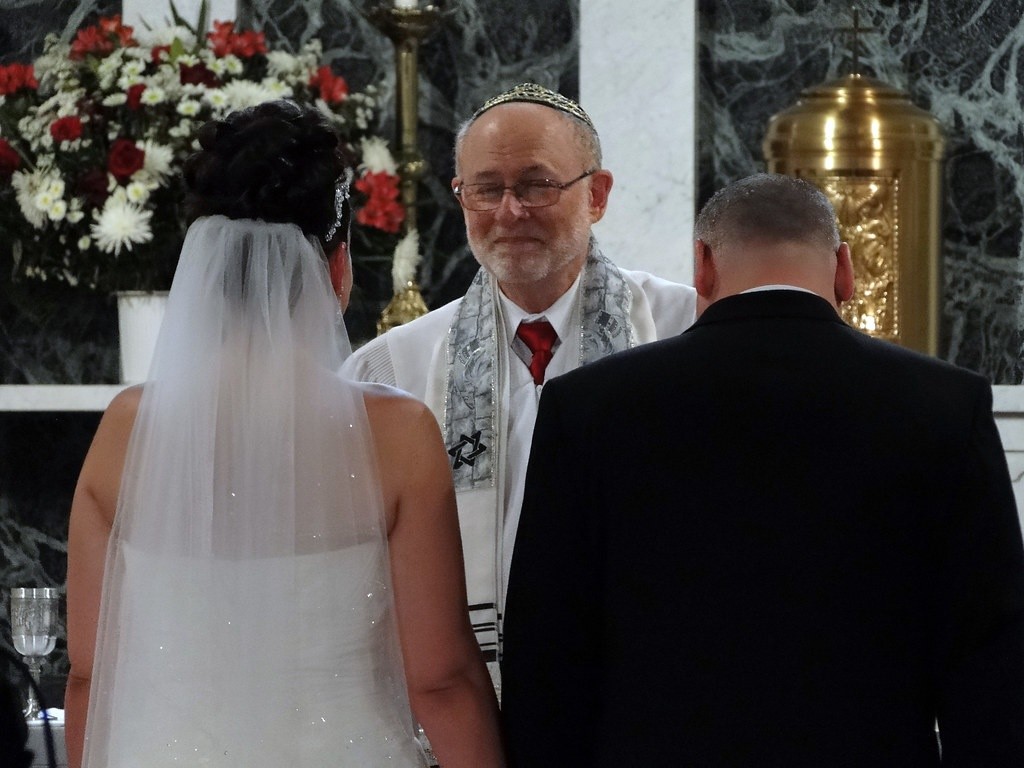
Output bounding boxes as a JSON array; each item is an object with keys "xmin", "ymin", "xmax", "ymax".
[{"xmin": 515, "ymin": 321, "xmax": 558, "ymax": 387}]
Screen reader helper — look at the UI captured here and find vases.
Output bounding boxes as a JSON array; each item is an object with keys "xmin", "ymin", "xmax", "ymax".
[{"xmin": 113, "ymin": 292, "xmax": 170, "ymax": 387}]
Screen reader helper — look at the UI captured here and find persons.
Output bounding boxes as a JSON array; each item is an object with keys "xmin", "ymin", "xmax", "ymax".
[
  {"xmin": 65, "ymin": 101, "xmax": 500, "ymax": 768},
  {"xmin": 501, "ymin": 171, "xmax": 1024, "ymax": 768},
  {"xmin": 339, "ymin": 86, "xmax": 696, "ymax": 766}
]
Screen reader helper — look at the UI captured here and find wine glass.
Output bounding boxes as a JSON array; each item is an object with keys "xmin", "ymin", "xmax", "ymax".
[{"xmin": 10, "ymin": 587, "xmax": 61, "ymax": 725}]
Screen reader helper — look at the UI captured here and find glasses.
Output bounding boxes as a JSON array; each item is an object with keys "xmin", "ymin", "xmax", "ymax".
[{"xmin": 452, "ymin": 169, "xmax": 594, "ymax": 211}]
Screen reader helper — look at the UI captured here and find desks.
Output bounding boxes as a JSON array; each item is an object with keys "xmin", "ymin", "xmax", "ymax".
[{"xmin": 23, "ymin": 708, "xmax": 69, "ymax": 768}]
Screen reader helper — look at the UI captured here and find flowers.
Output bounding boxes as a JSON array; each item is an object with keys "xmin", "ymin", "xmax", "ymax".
[{"xmin": 0, "ymin": 0, "xmax": 422, "ymax": 297}]
